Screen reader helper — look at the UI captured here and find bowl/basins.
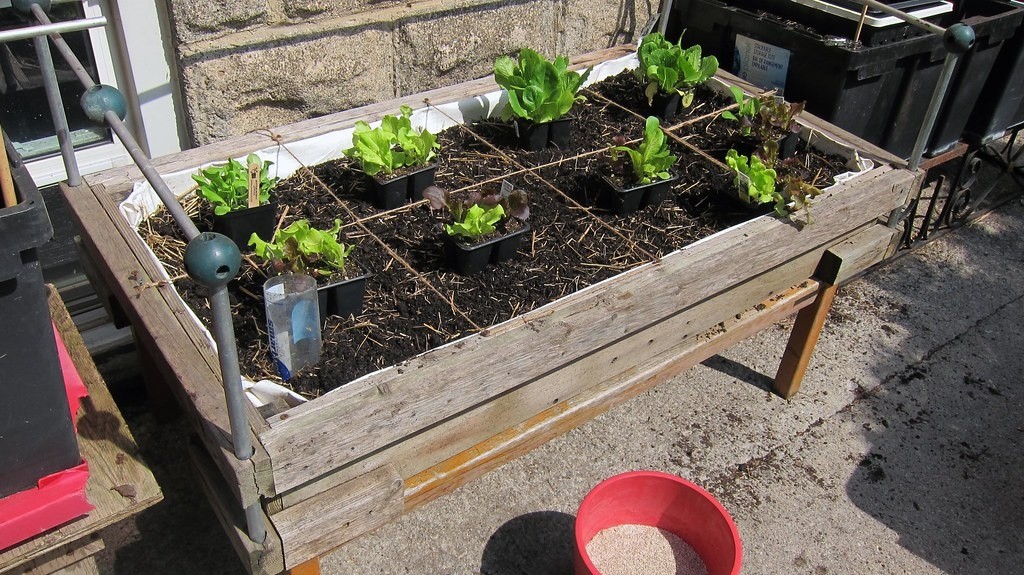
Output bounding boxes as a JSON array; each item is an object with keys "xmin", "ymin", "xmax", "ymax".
[{"xmin": 574, "ymin": 472, "xmax": 743, "ymax": 575}]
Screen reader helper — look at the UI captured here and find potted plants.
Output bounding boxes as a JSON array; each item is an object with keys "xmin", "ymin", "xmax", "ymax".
[
  {"xmin": 341, "ymin": 105, "xmax": 441, "ymax": 213},
  {"xmin": 716, "ymin": 148, "xmax": 826, "ymax": 228},
  {"xmin": 189, "ymin": 153, "xmax": 287, "ymax": 242},
  {"xmin": 422, "ymin": 178, "xmax": 532, "ymax": 279},
  {"xmin": 590, "ymin": 116, "xmax": 680, "ymax": 217},
  {"xmin": 718, "ymin": 84, "xmax": 808, "ymax": 162},
  {"xmin": 633, "ymin": 28, "xmax": 720, "ymax": 126},
  {"xmin": 493, "ymin": 47, "xmax": 594, "ymax": 153},
  {"xmin": 246, "ymin": 218, "xmax": 375, "ymax": 328}
]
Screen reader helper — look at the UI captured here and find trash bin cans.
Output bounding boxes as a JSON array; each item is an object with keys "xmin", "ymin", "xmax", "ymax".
[{"xmin": 656, "ymin": 0, "xmax": 1024, "ymax": 147}]
